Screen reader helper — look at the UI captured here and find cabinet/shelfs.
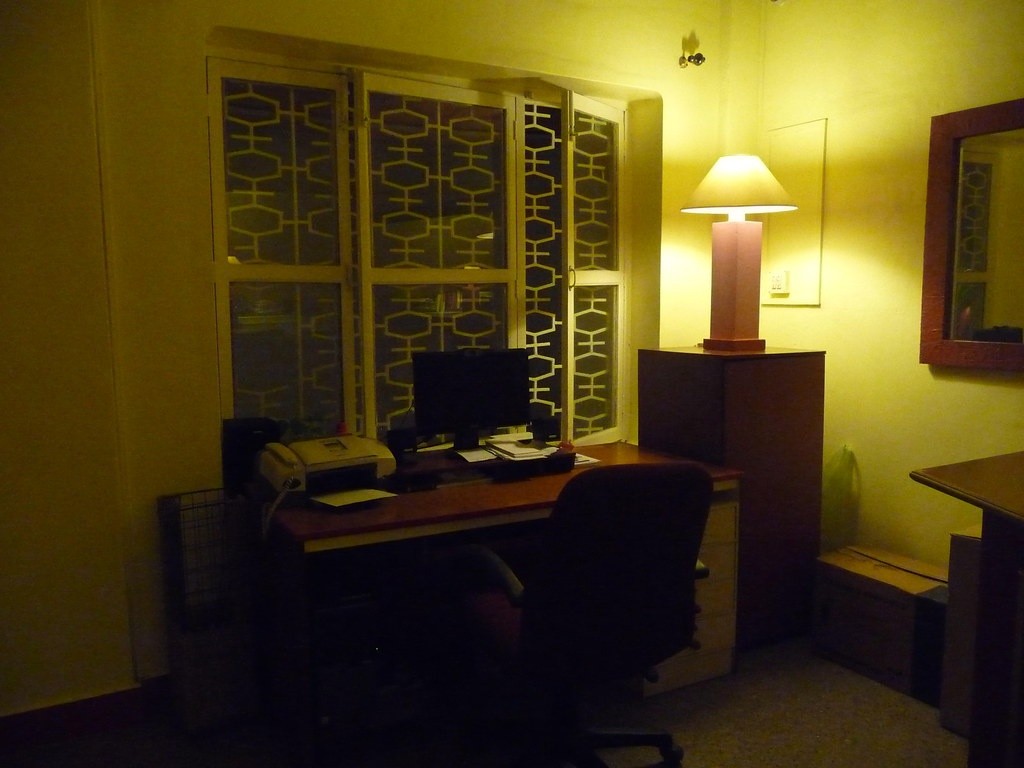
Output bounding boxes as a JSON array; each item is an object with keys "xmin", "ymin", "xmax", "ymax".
[{"xmin": 634, "ymin": 343, "xmax": 824, "ymax": 654}]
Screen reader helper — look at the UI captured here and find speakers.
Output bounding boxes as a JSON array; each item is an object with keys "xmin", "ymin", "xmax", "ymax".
[
  {"xmin": 385, "ymin": 429, "xmax": 417, "ymax": 464},
  {"xmin": 529, "ymin": 416, "xmax": 562, "ymax": 448}
]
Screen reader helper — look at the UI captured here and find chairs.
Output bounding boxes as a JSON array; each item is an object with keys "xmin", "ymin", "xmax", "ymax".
[{"xmin": 440, "ymin": 456, "xmax": 719, "ymax": 768}]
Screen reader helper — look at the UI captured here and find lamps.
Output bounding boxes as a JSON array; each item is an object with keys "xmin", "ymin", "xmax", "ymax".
[
  {"xmin": 678, "ymin": 150, "xmax": 803, "ymax": 349},
  {"xmin": 679, "ymin": 52, "xmax": 707, "ymax": 70}
]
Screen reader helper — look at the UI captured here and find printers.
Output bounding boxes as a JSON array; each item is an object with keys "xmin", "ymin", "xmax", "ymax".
[{"xmin": 262, "ymin": 436, "xmax": 397, "ymax": 509}]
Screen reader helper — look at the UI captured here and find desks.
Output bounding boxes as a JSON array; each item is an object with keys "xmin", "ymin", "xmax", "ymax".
[
  {"xmin": 903, "ymin": 448, "xmax": 1024, "ymax": 768},
  {"xmin": 259, "ymin": 438, "xmax": 745, "ymax": 768}
]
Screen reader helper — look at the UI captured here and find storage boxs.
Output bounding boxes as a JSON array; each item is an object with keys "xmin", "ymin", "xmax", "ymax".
[
  {"xmin": 811, "ymin": 539, "xmax": 950, "ymax": 697},
  {"xmin": 937, "ymin": 524, "xmax": 983, "ymax": 736},
  {"xmin": 164, "ymin": 604, "xmax": 263, "ymax": 735},
  {"xmin": 157, "ymin": 487, "xmax": 251, "ymax": 606}
]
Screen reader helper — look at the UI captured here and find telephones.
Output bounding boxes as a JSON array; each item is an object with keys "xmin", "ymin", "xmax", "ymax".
[{"xmin": 260, "ymin": 442, "xmax": 306, "ymax": 493}]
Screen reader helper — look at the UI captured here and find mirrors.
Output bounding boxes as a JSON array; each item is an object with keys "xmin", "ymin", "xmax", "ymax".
[{"xmin": 919, "ymin": 92, "xmax": 1024, "ymax": 375}]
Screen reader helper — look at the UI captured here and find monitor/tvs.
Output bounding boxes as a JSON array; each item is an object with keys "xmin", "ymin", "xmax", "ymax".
[{"xmin": 411, "ymin": 347, "xmax": 530, "ymax": 458}]
